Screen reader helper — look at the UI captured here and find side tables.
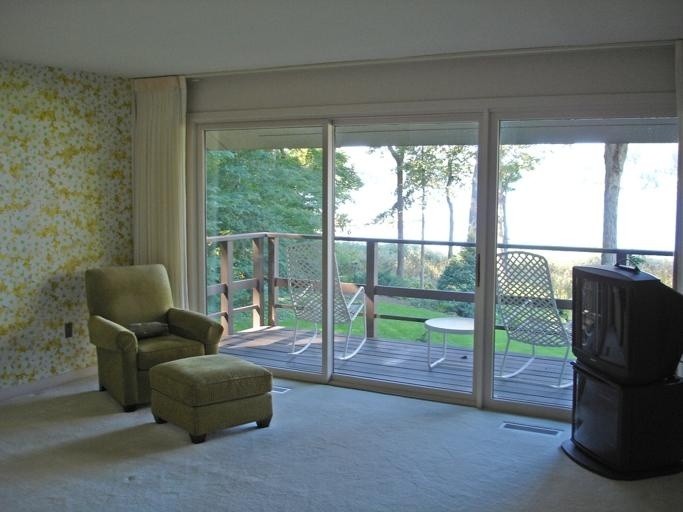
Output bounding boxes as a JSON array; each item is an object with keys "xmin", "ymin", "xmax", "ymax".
[{"xmin": 421, "ymin": 315, "xmax": 476, "ymax": 371}]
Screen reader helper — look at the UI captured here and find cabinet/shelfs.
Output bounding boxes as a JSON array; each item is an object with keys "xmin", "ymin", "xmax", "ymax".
[{"xmin": 554, "ymin": 361, "xmax": 682, "ymax": 483}]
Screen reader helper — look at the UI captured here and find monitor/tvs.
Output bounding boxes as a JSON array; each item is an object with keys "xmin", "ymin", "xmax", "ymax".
[{"xmin": 572, "ymin": 265, "xmax": 683, "ymax": 387}]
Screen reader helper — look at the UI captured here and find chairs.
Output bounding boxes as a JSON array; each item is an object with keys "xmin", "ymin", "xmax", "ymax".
[
  {"xmin": 279, "ymin": 238, "xmax": 369, "ymax": 362},
  {"xmin": 490, "ymin": 249, "xmax": 575, "ymax": 390},
  {"xmin": 82, "ymin": 262, "xmax": 225, "ymax": 412}
]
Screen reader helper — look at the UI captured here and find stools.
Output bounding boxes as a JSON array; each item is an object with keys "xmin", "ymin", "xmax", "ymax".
[{"xmin": 145, "ymin": 352, "xmax": 272, "ymax": 446}]
